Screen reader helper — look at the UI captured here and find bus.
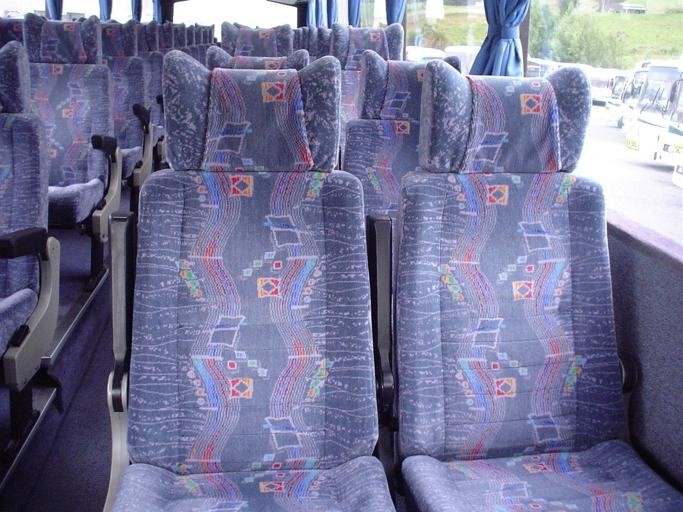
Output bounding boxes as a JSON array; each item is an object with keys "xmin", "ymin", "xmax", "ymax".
[
  {"xmin": 525, "ymin": 58, "xmax": 609, "ymax": 104},
  {"xmin": 601, "ymin": 55, "xmax": 683, "ymax": 192}
]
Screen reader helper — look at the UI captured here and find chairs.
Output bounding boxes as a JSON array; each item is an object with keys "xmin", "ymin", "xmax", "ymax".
[{"xmin": 1, "ymin": 12, "xmax": 683, "ymax": 506}]
